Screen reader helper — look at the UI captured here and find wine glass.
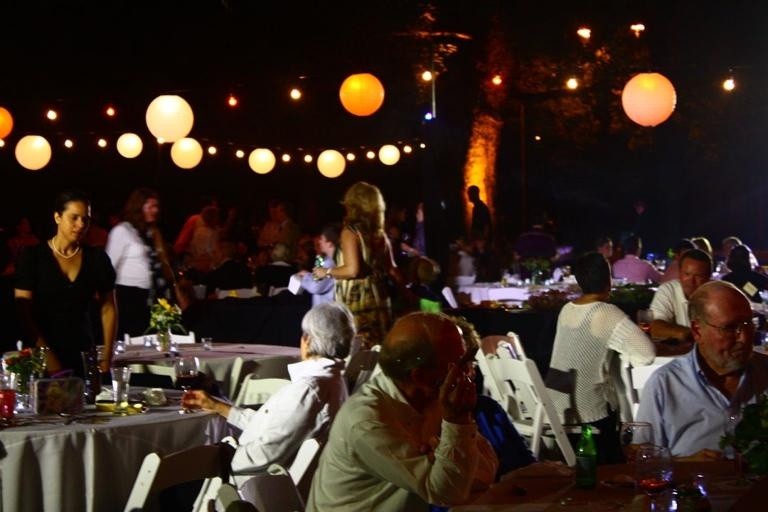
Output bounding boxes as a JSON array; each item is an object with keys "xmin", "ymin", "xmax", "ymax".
[
  {"xmin": 721, "ymin": 404, "xmax": 756, "ymax": 489},
  {"xmin": 616, "ymin": 420, "xmax": 708, "ymax": 512},
  {"xmin": 172, "ymin": 353, "xmax": 203, "ymax": 413}
]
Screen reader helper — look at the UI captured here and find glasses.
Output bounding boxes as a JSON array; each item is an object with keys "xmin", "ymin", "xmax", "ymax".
[{"xmin": 697, "ymin": 317, "xmax": 756, "ymax": 338}]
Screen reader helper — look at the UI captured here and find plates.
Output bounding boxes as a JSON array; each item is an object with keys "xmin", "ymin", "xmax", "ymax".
[{"xmin": 506, "ymin": 309, "xmax": 527, "ymax": 314}]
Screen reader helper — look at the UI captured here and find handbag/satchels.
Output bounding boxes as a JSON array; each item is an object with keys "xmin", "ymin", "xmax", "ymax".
[
  {"xmin": 334, "ymin": 223, "xmax": 386, "ymax": 313},
  {"xmin": 152, "ymin": 226, "xmax": 198, "ymax": 313}
]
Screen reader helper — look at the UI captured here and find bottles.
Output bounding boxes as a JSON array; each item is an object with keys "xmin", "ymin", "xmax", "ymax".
[
  {"xmin": 0, "ymin": 389, "xmax": 15, "ymax": 415},
  {"xmin": 312, "ymin": 254, "xmax": 324, "ymax": 282},
  {"xmin": 81, "ymin": 344, "xmax": 106, "ymax": 404},
  {"xmin": 575, "ymin": 422, "xmax": 598, "ymax": 490}
]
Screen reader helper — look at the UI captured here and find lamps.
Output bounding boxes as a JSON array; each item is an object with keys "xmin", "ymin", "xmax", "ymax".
[{"xmin": 0, "ymin": 2, "xmax": 679, "ymax": 179}]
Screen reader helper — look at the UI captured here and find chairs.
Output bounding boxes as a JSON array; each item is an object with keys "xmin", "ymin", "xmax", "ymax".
[
  {"xmin": 120, "ymin": 435, "xmax": 327, "ymax": 510},
  {"xmin": 617, "ymin": 351, "xmax": 679, "ymax": 423},
  {"xmin": 475, "ymin": 349, "xmax": 581, "ymax": 469},
  {"xmin": 1, "ymin": 254, "xmax": 767, "ymax": 399}
]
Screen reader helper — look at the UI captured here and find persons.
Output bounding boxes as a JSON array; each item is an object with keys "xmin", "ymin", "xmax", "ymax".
[
  {"xmin": 383, "ymin": 185, "xmax": 558, "ymax": 286},
  {"xmin": 547, "ymin": 233, "xmax": 767, "ymax": 463},
  {"xmin": 172, "ymin": 195, "xmax": 343, "ymax": 312},
  {"xmin": 2, "ymin": 187, "xmax": 190, "ymax": 386},
  {"xmin": 302, "ymin": 310, "xmax": 500, "ymax": 512},
  {"xmin": 181, "ymin": 303, "xmax": 355, "ymax": 483},
  {"xmin": 452, "ymin": 317, "xmax": 537, "ymax": 483},
  {"xmin": 289, "ymin": 182, "xmax": 400, "ymax": 350}
]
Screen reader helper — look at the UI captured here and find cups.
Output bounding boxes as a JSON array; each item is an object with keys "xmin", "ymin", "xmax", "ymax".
[
  {"xmin": 636, "ymin": 306, "xmax": 655, "ymax": 336},
  {"xmin": 109, "ymin": 363, "xmax": 134, "ymax": 407},
  {"xmin": 113, "ymin": 336, "xmax": 213, "ymax": 355}
]
